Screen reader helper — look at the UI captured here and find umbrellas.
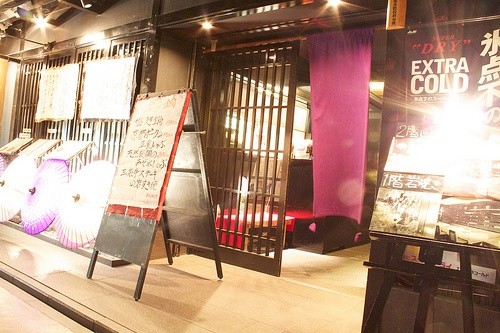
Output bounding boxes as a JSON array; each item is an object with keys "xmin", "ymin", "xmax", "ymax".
[
  {"xmin": 56, "ymin": 159, "xmax": 118, "ymax": 251},
  {"xmin": 21, "ymin": 159, "xmax": 69, "ymax": 235},
  {"xmin": 0, "ymin": 155, "xmax": 36, "ymax": 224}
]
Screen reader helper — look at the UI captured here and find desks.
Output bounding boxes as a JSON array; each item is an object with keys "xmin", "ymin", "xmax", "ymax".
[{"xmin": 215, "ymin": 209, "xmax": 319, "ymax": 253}]
